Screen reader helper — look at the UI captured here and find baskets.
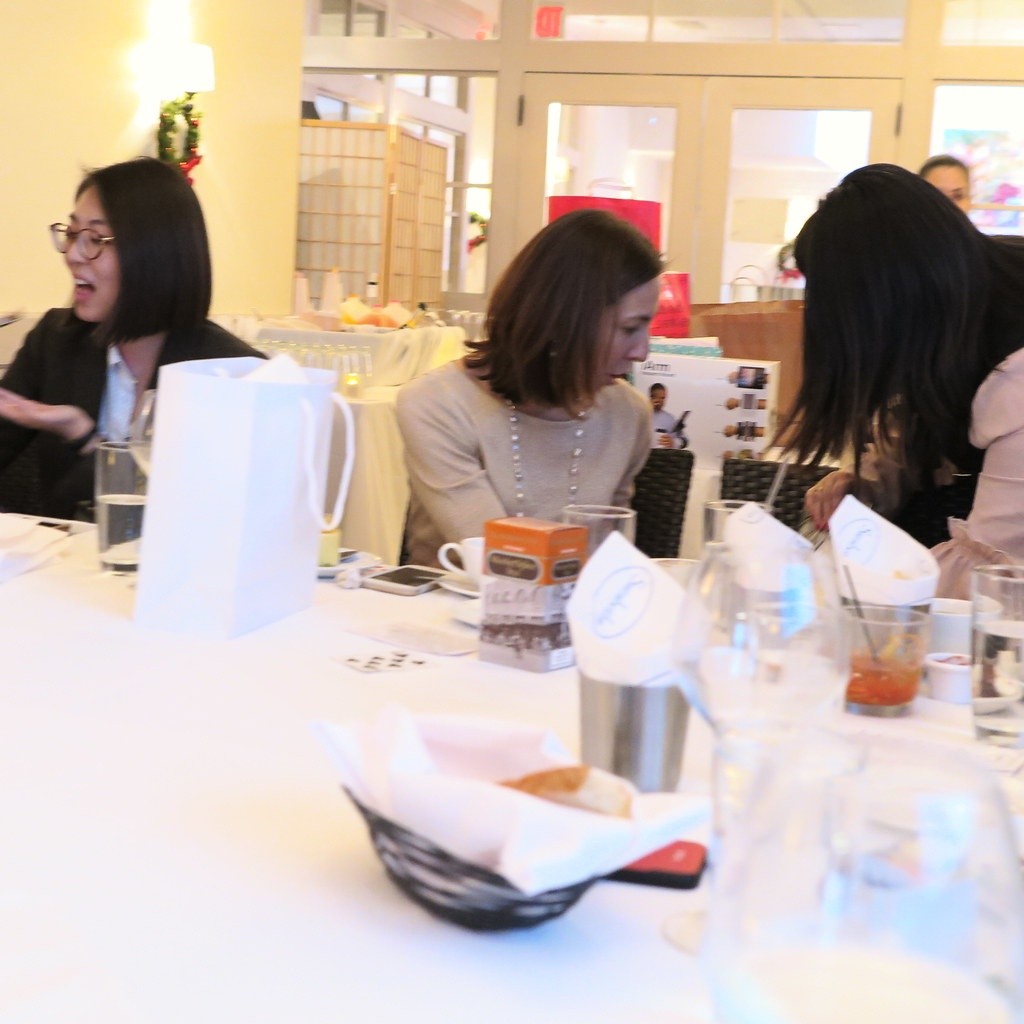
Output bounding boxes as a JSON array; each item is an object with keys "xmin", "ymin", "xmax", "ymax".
[{"xmin": 341, "ymin": 789, "xmax": 599, "ymax": 930}]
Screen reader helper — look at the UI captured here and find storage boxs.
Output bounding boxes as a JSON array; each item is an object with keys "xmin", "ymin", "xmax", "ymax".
[
  {"xmin": 477, "ymin": 514, "xmax": 590, "ymax": 675},
  {"xmin": 649, "ymin": 273, "xmax": 689, "ymax": 338},
  {"xmin": 547, "ymin": 196, "xmax": 661, "ymax": 250},
  {"xmin": 631, "ymin": 353, "xmax": 781, "ymax": 472}
]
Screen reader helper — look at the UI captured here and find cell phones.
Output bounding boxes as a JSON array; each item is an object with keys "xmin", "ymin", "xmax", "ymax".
[
  {"xmin": 601, "ymin": 841, "xmax": 710, "ymax": 889},
  {"xmin": 363, "ymin": 564, "xmax": 452, "ymax": 596},
  {"xmin": 36, "ymin": 522, "xmax": 69, "ymax": 530}
]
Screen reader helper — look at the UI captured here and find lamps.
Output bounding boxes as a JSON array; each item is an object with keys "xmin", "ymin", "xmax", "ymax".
[{"xmin": 122, "ymin": 38, "xmax": 215, "ymax": 96}]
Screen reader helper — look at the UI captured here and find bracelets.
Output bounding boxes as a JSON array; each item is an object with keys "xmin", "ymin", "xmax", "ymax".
[{"xmin": 62, "ymin": 425, "xmax": 99, "ymax": 454}]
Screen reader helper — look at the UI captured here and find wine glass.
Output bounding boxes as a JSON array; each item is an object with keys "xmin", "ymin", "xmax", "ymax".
[{"xmin": 661, "ymin": 552, "xmax": 855, "ymax": 961}]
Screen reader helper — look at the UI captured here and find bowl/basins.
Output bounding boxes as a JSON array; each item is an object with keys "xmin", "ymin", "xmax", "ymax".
[{"xmin": 925, "ymin": 652, "xmax": 972, "ymax": 703}]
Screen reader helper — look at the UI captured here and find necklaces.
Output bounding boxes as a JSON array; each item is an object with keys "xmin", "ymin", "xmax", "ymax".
[{"xmin": 506, "ymin": 397, "xmax": 585, "ymax": 518}]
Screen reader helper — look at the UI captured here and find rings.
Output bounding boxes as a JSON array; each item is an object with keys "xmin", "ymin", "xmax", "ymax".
[{"xmin": 814, "ymin": 485, "xmax": 823, "ymax": 491}]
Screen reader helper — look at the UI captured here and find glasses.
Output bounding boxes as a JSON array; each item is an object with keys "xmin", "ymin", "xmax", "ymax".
[{"xmin": 51, "ymin": 223, "xmax": 119, "ymax": 262}]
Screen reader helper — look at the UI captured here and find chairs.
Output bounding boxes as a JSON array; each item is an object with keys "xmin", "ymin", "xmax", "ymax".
[
  {"xmin": 630, "ymin": 449, "xmax": 695, "ymax": 559},
  {"xmin": 721, "ymin": 456, "xmax": 841, "ymax": 532}
]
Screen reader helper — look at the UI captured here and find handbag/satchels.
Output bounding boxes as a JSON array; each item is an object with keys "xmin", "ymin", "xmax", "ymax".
[{"xmin": 131, "ymin": 354, "xmax": 356, "ymax": 638}]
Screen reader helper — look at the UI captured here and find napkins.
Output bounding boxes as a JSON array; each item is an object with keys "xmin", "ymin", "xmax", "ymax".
[
  {"xmin": 563, "ymin": 528, "xmax": 707, "ymax": 689},
  {"xmin": 719, "ymin": 501, "xmax": 817, "ymax": 636},
  {"xmin": 343, "ymin": 703, "xmax": 706, "ymax": 899},
  {"xmin": 825, "ymin": 492, "xmax": 943, "ymax": 605}
]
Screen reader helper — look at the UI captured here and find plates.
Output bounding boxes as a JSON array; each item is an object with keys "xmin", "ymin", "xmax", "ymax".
[
  {"xmin": 317, "ymin": 547, "xmax": 383, "ymax": 577},
  {"xmin": 436, "ymin": 573, "xmax": 483, "ymax": 599}
]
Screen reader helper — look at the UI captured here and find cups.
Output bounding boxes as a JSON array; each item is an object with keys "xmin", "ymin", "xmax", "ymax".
[
  {"xmin": 561, "ymin": 504, "xmax": 638, "ymax": 563},
  {"xmin": 842, "ymin": 605, "xmax": 933, "ymax": 720},
  {"xmin": 702, "ymin": 499, "xmax": 776, "ymax": 544},
  {"xmin": 93, "ymin": 439, "xmax": 153, "ymax": 576},
  {"xmin": 440, "ymin": 536, "xmax": 486, "ymax": 587},
  {"xmin": 969, "ymin": 564, "xmax": 1024, "ymax": 721},
  {"xmin": 930, "ymin": 598, "xmax": 972, "ymax": 655},
  {"xmin": 580, "ymin": 668, "xmax": 690, "ymax": 794}
]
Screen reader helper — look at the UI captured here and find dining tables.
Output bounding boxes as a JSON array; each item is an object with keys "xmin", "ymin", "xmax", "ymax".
[
  {"xmin": 0, "ymin": 510, "xmax": 1024, "ymax": 1024},
  {"xmin": 323, "ymin": 387, "xmax": 409, "ymax": 566}
]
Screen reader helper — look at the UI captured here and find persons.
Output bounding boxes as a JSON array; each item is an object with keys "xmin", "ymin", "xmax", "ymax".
[
  {"xmin": 392, "ymin": 208, "xmax": 666, "ymax": 575},
  {"xmin": 649, "ymin": 383, "xmax": 689, "ymax": 450},
  {"xmin": 0, "ymin": 157, "xmax": 268, "ymax": 523},
  {"xmin": 774, "ymin": 164, "xmax": 1023, "ymax": 601},
  {"xmin": 920, "ymin": 155, "xmax": 971, "ymax": 217}
]
